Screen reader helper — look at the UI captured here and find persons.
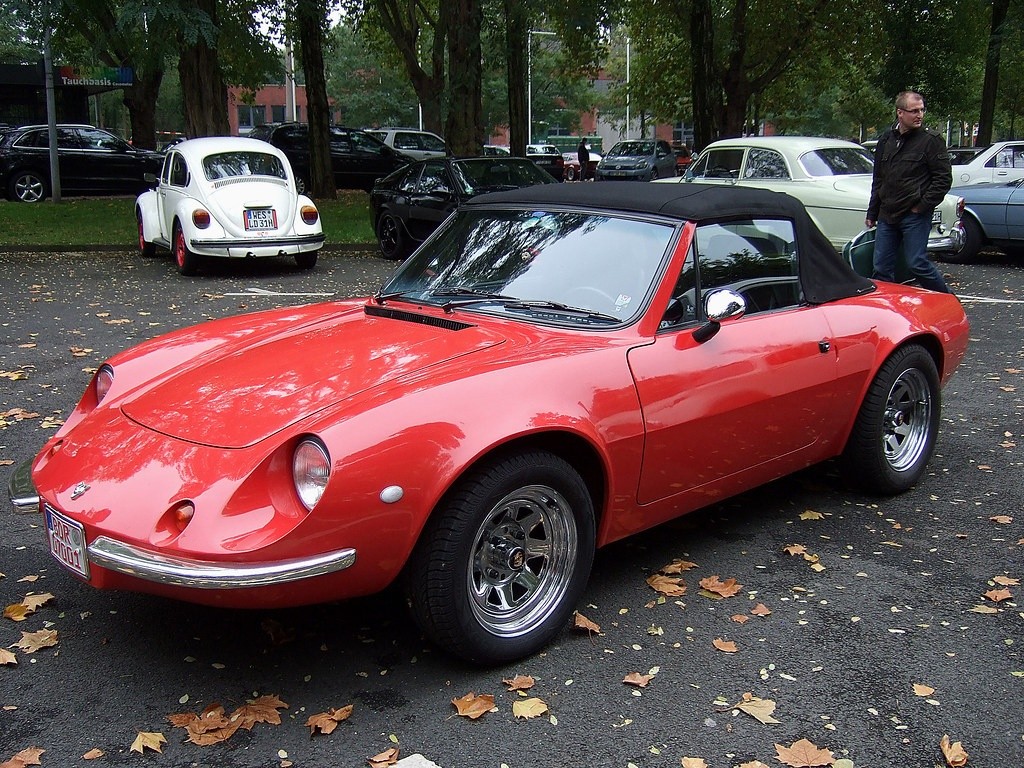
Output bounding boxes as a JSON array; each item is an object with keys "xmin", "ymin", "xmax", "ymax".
[
  {"xmin": 864, "ymin": 92, "xmax": 963, "ymax": 304},
  {"xmin": 577, "ymin": 137, "xmax": 589, "ymax": 181}
]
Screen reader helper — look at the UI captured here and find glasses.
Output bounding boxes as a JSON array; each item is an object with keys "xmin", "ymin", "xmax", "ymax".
[{"xmin": 899, "ymin": 107, "xmax": 926, "ymax": 113}]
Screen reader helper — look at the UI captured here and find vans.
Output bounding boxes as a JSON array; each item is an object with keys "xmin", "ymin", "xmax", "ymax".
[{"xmin": 247, "ymin": 121, "xmax": 418, "ymax": 196}]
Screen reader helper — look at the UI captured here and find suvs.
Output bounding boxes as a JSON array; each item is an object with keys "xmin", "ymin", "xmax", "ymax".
[{"xmin": 0, "ymin": 123, "xmax": 167, "ymax": 204}]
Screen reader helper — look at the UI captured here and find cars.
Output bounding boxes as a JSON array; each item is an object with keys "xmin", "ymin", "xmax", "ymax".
[
  {"xmin": 8, "ymin": 180, "xmax": 969, "ymax": 669},
  {"xmin": 595, "ymin": 138, "xmax": 679, "ymax": 181},
  {"xmin": 935, "ymin": 178, "xmax": 1024, "ymax": 264},
  {"xmin": 526, "ymin": 144, "xmax": 564, "ymax": 183},
  {"xmin": 368, "ymin": 157, "xmax": 560, "ymax": 260},
  {"xmin": 951, "ymin": 141, "xmax": 1024, "ymax": 188},
  {"xmin": 670, "ymin": 145, "xmax": 693, "ymax": 175},
  {"xmin": 561, "ymin": 153, "xmax": 602, "ymax": 181},
  {"xmin": 649, "ymin": 136, "xmax": 966, "ymax": 253},
  {"xmin": 364, "ymin": 130, "xmax": 446, "ymax": 161},
  {"xmin": 947, "ymin": 144, "xmax": 986, "ymax": 165},
  {"xmin": 134, "ymin": 137, "xmax": 327, "ymax": 277}
]
